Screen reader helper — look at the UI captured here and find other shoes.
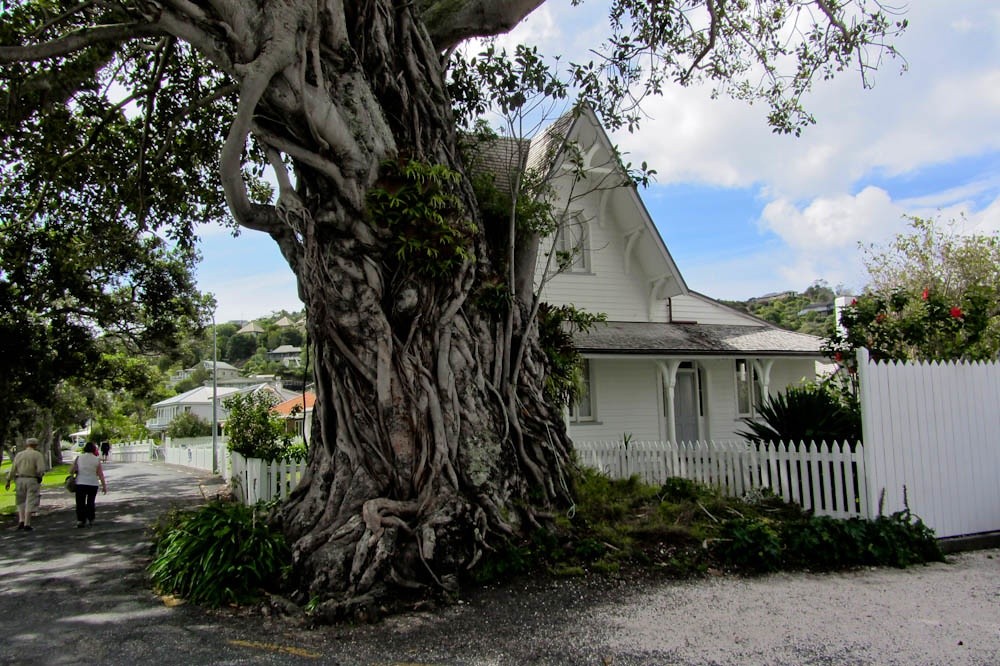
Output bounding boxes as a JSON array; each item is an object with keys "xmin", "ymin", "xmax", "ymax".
[
  {"xmin": 24, "ymin": 525, "xmax": 33, "ymax": 531},
  {"xmin": 17, "ymin": 522, "xmax": 24, "ymax": 529}
]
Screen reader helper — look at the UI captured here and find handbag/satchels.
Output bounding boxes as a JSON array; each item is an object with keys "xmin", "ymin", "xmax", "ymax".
[
  {"xmin": 37, "ymin": 474, "xmax": 42, "ymax": 483},
  {"xmin": 65, "ymin": 455, "xmax": 79, "ymax": 492}
]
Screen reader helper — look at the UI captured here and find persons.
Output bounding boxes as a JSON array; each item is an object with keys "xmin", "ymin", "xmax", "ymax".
[
  {"xmin": 101, "ymin": 439, "xmax": 110, "ymax": 464},
  {"xmin": 91, "ymin": 440, "xmax": 100, "ymax": 457},
  {"xmin": 4, "ymin": 437, "xmax": 45, "ymax": 535},
  {"xmin": 70, "ymin": 442, "xmax": 107, "ymax": 529}
]
